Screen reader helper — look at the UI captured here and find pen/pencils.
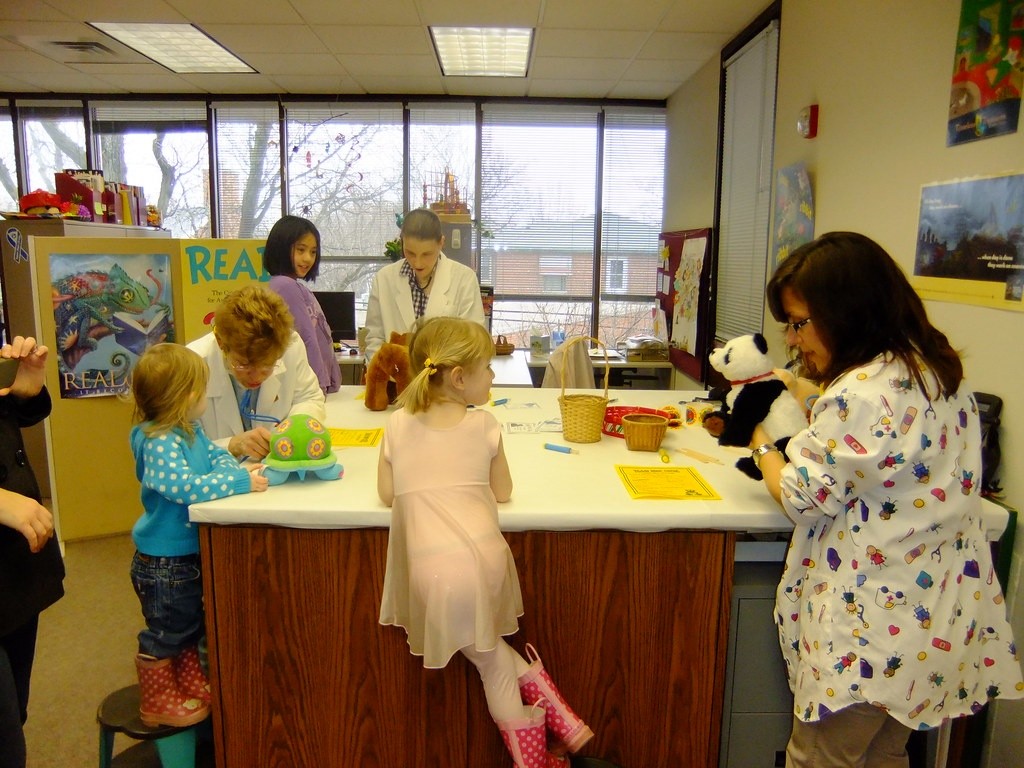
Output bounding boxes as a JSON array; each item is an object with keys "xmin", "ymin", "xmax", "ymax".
[{"xmin": 608, "ymin": 398, "xmax": 619, "ymax": 403}]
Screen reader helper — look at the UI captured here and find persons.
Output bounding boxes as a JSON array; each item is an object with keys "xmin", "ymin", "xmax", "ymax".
[
  {"xmin": 746, "ymin": 230, "xmax": 1024, "ymax": 768},
  {"xmin": 0, "ymin": 335, "xmax": 68, "ymax": 768},
  {"xmin": 261, "ymin": 214, "xmax": 343, "ymax": 404},
  {"xmin": 114, "ymin": 342, "xmax": 269, "ymax": 729},
  {"xmin": 363, "ymin": 207, "xmax": 489, "ymax": 386},
  {"xmin": 376, "ymin": 315, "xmax": 596, "ymax": 768},
  {"xmin": 148, "ymin": 283, "xmax": 328, "ymax": 749}
]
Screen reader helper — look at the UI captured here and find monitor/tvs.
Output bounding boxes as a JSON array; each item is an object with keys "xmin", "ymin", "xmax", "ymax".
[{"xmin": 312, "ymin": 292, "xmax": 355, "ymax": 352}]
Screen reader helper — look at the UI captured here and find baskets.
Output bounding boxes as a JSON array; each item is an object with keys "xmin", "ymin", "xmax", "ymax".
[
  {"xmin": 601, "ymin": 405, "xmax": 668, "ymax": 438},
  {"xmin": 557, "ymin": 336, "xmax": 610, "ymax": 444},
  {"xmin": 621, "ymin": 414, "xmax": 669, "ymax": 452}
]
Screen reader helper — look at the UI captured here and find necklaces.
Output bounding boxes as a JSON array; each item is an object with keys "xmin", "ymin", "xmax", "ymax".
[{"xmin": 413, "ymin": 261, "xmax": 438, "ymax": 308}]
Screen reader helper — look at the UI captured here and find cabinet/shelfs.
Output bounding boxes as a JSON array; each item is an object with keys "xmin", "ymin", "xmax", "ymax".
[
  {"xmin": 716, "ymin": 561, "xmax": 790, "ymax": 768},
  {"xmin": 0, "ymin": 218, "xmax": 173, "ymax": 501}
]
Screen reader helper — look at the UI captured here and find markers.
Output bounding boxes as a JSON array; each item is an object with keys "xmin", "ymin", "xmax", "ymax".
[
  {"xmin": 660, "ymin": 449, "xmax": 670, "ymax": 463},
  {"xmin": 490, "ymin": 398, "xmax": 511, "ymax": 407},
  {"xmin": 542, "ymin": 443, "xmax": 578, "ymax": 455}
]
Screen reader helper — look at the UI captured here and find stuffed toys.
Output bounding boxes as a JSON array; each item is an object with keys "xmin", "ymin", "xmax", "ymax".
[
  {"xmin": 698, "ymin": 331, "xmax": 828, "ymax": 483},
  {"xmin": 363, "ymin": 330, "xmax": 417, "ymax": 411},
  {"xmin": 256, "ymin": 413, "xmax": 345, "ymax": 487}
]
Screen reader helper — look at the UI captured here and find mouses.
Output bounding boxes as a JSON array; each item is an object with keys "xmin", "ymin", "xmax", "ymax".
[{"xmin": 349, "ymin": 349, "xmax": 357, "ymax": 355}]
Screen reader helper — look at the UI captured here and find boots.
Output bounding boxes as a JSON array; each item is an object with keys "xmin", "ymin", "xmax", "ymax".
[
  {"xmin": 135, "ymin": 644, "xmax": 213, "ymax": 729},
  {"xmin": 516, "ymin": 642, "xmax": 594, "ymax": 756},
  {"xmin": 486, "ymin": 697, "xmax": 571, "ymax": 768}
]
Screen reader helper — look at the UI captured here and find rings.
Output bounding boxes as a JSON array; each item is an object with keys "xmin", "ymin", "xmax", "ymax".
[{"xmin": 30, "ymin": 345, "xmax": 38, "ymax": 354}]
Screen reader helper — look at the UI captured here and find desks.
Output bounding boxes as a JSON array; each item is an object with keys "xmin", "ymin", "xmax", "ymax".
[
  {"xmin": 333, "ymin": 348, "xmax": 676, "ymax": 390},
  {"xmin": 188, "ymin": 387, "xmax": 792, "ymax": 768}
]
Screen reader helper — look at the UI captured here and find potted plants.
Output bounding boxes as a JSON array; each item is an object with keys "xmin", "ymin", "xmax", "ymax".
[{"xmin": 384, "ymin": 239, "xmax": 403, "ymax": 263}]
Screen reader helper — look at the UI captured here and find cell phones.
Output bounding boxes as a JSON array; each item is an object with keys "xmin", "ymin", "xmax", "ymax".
[{"xmin": 0, "ymin": 357, "xmax": 21, "ymax": 390}]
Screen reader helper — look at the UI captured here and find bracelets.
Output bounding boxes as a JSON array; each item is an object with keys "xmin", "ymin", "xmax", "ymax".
[{"xmin": 752, "ymin": 442, "xmax": 779, "ymax": 472}]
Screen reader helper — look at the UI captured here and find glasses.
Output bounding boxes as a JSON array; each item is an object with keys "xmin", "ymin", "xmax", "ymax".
[
  {"xmin": 787, "ymin": 316, "xmax": 812, "ymax": 334},
  {"xmin": 221, "ymin": 343, "xmax": 280, "ymax": 372}
]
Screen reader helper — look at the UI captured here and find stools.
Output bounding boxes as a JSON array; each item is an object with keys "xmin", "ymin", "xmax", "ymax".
[{"xmin": 96, "ymin": 684, "xmax": 214, "ymax": 768}]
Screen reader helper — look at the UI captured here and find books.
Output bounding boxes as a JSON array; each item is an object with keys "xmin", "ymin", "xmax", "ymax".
[{"xmin": 61, "ymin": 169, "xmax": 146, "ymax": 227}]
